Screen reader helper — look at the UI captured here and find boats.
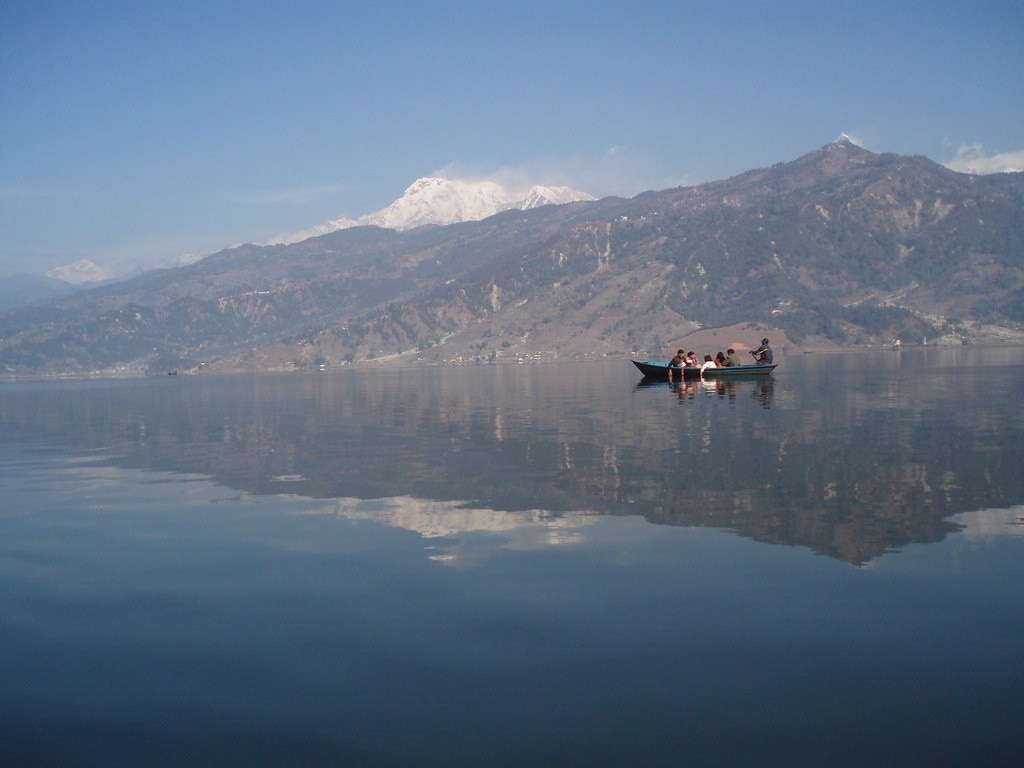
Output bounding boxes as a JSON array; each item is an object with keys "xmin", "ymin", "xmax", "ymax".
[{"xmin": 630, "ymin": 357, "xmax": 779, "ymax": 379}]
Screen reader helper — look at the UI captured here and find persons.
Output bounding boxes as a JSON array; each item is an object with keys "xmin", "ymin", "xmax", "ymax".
[
  {"xmin": 700, "ymin": 356, "xmax": 716, "ymax": 380},
  {"xmin": 721, "ymin": 349, "xmax": 740, "ymax": 367},
  {"xmin": 749, "ymin": 338, "xmax": 772, "ymax": 364},
  {"xmin": 714, "ymin": 352, "xmax": 725, "ymax": 367},
  {"xmin": 686, "ymin": 351, "xmax": 700, "ymax": 367},
  {"xmin": 668, "ymin": 349, "xmax": 686, "ymax": 383}
]
